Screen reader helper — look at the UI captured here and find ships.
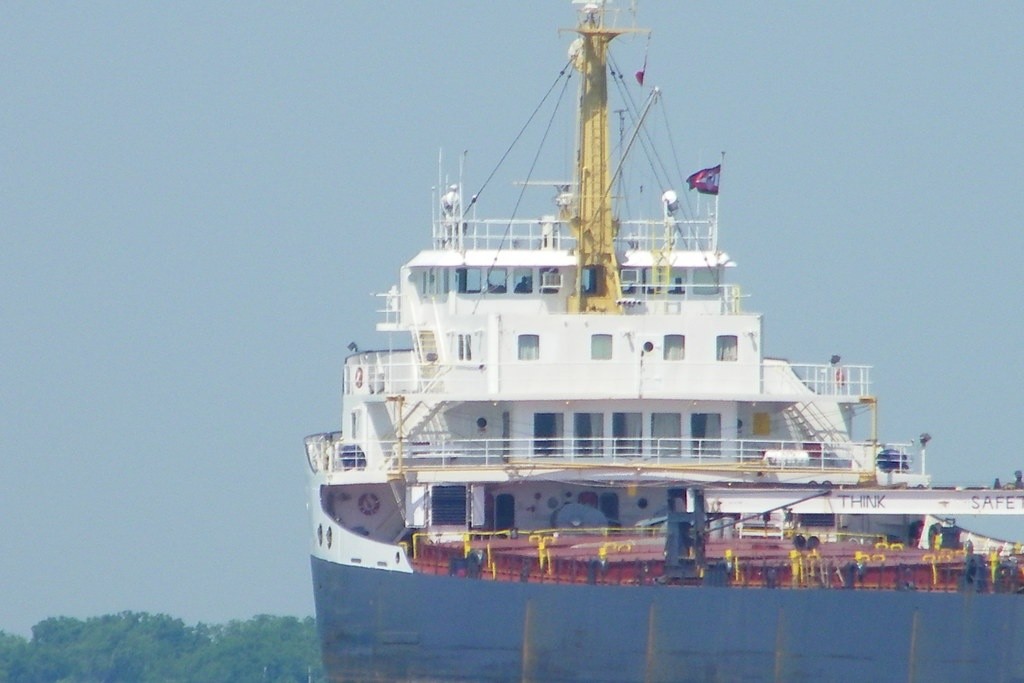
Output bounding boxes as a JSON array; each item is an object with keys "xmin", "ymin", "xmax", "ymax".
[{"xmin": 298, "ymin": 0, "xmax": 1024, "ymax": 683}]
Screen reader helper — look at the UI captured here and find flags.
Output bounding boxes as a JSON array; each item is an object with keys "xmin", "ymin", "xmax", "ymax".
[{"xmin": 684, "ymin": 164, "xmax": 719, "ymax": 196}]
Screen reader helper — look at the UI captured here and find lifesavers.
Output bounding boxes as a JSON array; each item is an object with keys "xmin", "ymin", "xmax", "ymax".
[
  {"xmin": 836, "ymin": 371, "xmax": 846, "ymax": 386},
  {"xmin": 358, "ymin": 493, "xmax": 379, "ymax": 516},
  {"xmin": 355, "ymin": 368, "xmax": 363, "ymax": 388}
]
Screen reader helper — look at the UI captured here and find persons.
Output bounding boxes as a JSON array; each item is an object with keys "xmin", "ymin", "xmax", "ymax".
[
  {"xmin": 666, "ymin": 522, "xmax": 699, "ymax": 558},
  {"xmin": 514, "ymin": 274, "xmax": 529, "ymax": 293}
]
